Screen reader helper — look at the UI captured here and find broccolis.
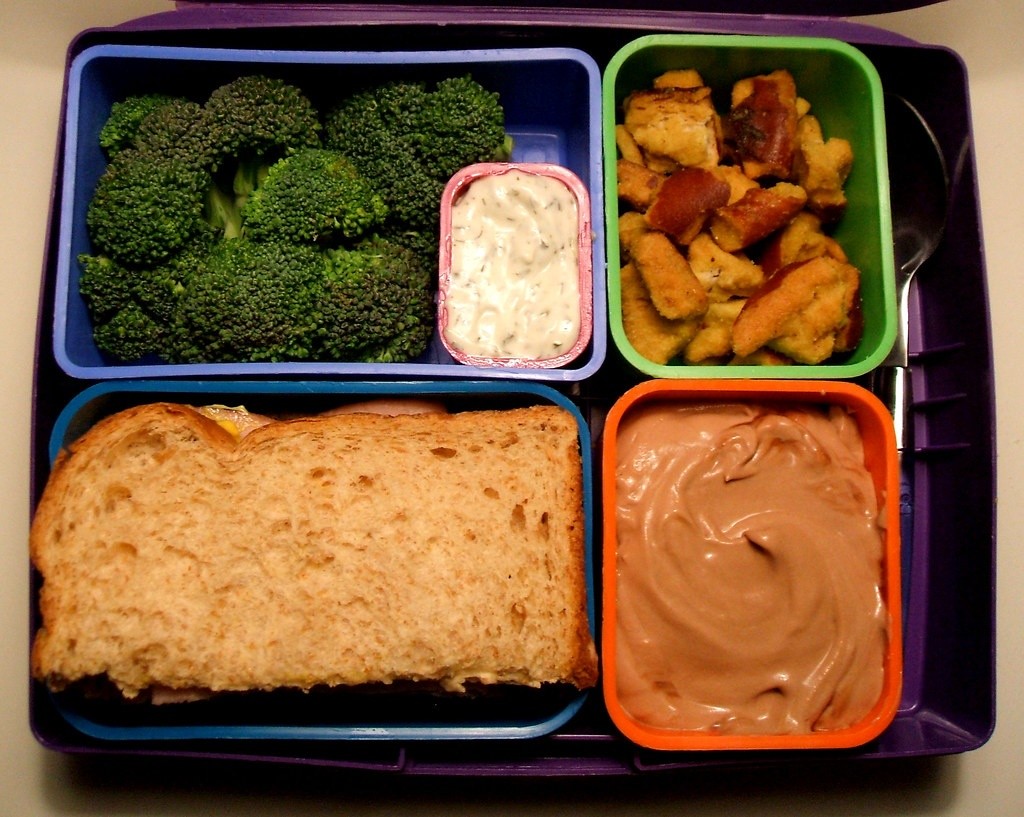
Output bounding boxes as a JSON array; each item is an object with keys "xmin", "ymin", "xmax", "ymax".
[{"xmin": 75, "ymin": 71, "xmax": 513, "ymax": 363}]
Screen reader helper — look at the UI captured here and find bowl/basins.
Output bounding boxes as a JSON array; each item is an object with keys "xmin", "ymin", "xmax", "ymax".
[{"xmin": 438, "ymin": 164, "xmax": 592, "ymax": 369}]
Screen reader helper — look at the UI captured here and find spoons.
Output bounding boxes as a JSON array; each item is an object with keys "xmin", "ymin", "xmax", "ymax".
[{"xmin": 880, "ymin": 93, "xmax": 953, "ymax": 496}]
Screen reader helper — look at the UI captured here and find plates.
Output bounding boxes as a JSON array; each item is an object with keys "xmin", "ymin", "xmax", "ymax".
[
  {"xmin": 602, "ymin": 34, "xmax": 900, "ymax": 380},
  {"xmin": 48, "ymin": 380, "xmax": 594, "ymax": 737},
  {"xmin": 52, "ymin": 44, "xmax": 605, "ymax": 381},
  {"xmin": 603, "ymin": 382, "xmax": 903, "ymax": 752}
]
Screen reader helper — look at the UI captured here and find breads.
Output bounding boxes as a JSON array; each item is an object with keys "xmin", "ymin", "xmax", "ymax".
[{"xmin": 28, "ymin": 397, "xmax": 601, "ymax": 706}]
